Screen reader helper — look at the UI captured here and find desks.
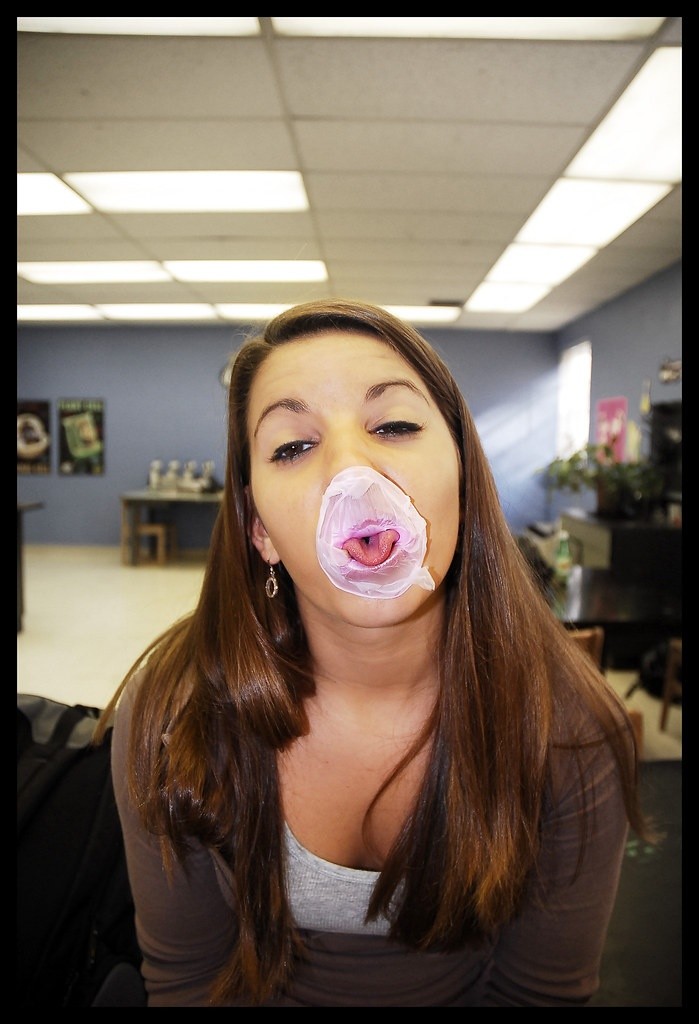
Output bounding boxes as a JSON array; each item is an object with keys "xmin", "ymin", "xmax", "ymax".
[
  {"xmin": 119, "ymin": 485, "xmax": 226, "ymax": 563},
  {"xmin": 536, "ymin": 582, "xmax": 682, "ymax": 675}
]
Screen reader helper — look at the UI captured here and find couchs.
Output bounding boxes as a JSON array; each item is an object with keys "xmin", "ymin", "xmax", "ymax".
[{"xmin": 15, "ymin": 693, "xmax": 681, "ymax": 1008}]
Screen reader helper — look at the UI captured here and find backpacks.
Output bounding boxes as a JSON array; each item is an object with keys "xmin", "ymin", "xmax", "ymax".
[{"xmin": 17, "ymin": 698, "xmax": 150, "ymax": 1007}]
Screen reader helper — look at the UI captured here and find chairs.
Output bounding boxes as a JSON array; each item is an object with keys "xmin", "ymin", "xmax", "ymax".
[{"xmin": 122, "ymin": 497, "xmax": 176, "ymax": 566}]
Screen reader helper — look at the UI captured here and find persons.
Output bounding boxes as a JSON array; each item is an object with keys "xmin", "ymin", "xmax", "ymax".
[{"xmin": 92, "ymin": 300, "xmax": 666, "ymax": 1005}]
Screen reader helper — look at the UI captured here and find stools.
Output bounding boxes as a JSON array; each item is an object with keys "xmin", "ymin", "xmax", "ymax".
[{"xmin": 661, "ymin": 638, "xmax": 684, "ymax": 730}]
